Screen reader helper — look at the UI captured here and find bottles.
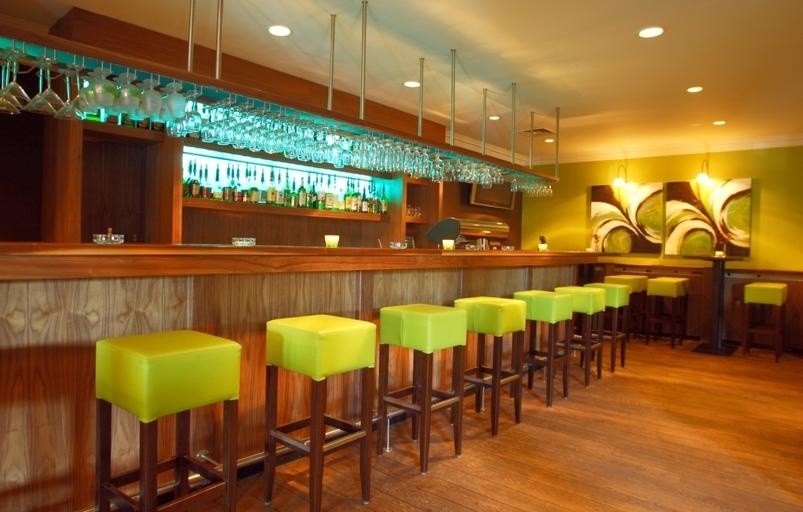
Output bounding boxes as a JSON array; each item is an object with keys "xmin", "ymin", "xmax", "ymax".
[
  {"xmin": 275, "ymin": 174, "xmax": 284, "ymax": 208},
  {"xmin": 190, "ymin": 159, "xmax": 200, "ymax": 198},
  {"xmin": 182, "ymin": 159, "xmax": 192, "ymax": 197},
  {"xmin": 368, "ymin": 181, "xmax": 373, "ymax": 213},
  {"xmin": 284, "ymin": 168, "xmax": 290, "ymax": 207},
  {"xmin": 352, "ymin": 183, "xmax": 358, "ymax": 211},
  {"xmin": 231, "ymin": 164, "xmax": 236, "ymax": 192},
  {"xmin": 267, "ymin": 167, "xmax": 276, "ymax": 205},
  {"xmin": 362, "ymin": 188, "xmax": 368, "ymax": 213},
  {"xmin": 312, "ymin": 181, "xmax": 317, "ymax": 209},
  {"xmin": 376, "ymin": 191, "xmax": 382, "ymax": 214},
  {"xmin": 198, "ymin": 164, "xmax": 203, "ymax": 185},
  {"xmin": 373, "ymin": 182, "xmax": 379, "ymax": 214},
  {"xmin": 290, "ymin": 177, "xmax": 298, "ymax": 209},
  {"xmin": 333, "ymin": 175, "xmax": 338, "ymax": 211},
  {"xmin": 380, "ymin": 183, "xmax": 389, "ymax": 214},
  {"xmin": 318, "ymin": 174, "xmax": 325, "ymax": 209},
  {"xmin": 248, "ymin": 168, "xmax": 253, "ymax": 203},
  {"xmin": 315, "ymin": 174, "xmax": 320, "ymax": 209},
  {"xmin": 298, "ymin": 177, "xmax": 306, "ymax": 208},
  {"xmin": 338, "ymin": 187, "xmax": 345, "ymax": 212},
  {"xmin": 251, "ymin": 165, "xmax": 259, "ymax": 203},
  {"xmin": 222, "ymin": 163, "xmax": 232, "ymax": 201},
  {"xmin": 182, "ymin": 168, "xmax": 186, "ymax": 184},
  {"xmin": 242, "ymin": 163, "xmax": 249, "ymax": 202},
  {"xmin": 258, "ymin": 169, "xmax": 267, "ymax": 203},
  {"xmin": 357, "ymin": 179, "xmax": 362, "ymax": 212},
  {"xmin": 343, "ymin": 180, "xmax": 352, "ymax": 211},
  {"xmin": 234, "ymin": 165, "xmax": 242, "ymax": 202},
  {"xmin": 199, "ymin": 163, "xmax": 211, "ymax": 200},
  {"xmin": 325, "ymin": 175, "xmax": 333, "ymax": 210},
  {"xmin": 306, "ymin": 176, "xmax": 312, "ymax": 208},
  {"xmin": 212, "ymin": 163, "xmax": 222, "ymax": 201}
]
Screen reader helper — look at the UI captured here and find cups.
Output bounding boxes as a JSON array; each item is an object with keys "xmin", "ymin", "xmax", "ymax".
[
  {"xmin": 324, "ymin": 235, "xmax": 340, "ymax": 248},
  {"xmin": 538, "ymin": 244, "xmax": 548, "ymax": 251},
  {"xmin": 443, "ymin": 240, "xmax": 454, "ymax": 250}
]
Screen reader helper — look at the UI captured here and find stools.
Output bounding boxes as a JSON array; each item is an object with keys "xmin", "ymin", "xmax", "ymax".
[
  {"xmin": 95, "ymin": 330, "xmax": 243, "ymax": 512},
  {"xmin": 743, "ymin": 281, "xmax": 788, "ymax": 363},
  {"xmin": 264, "ymin": 314, "xmax": 376, "ymax": 511},
  {"xmin": 646, "ymin": 276, "xmax": 690, "ymax": 349},
  {"xmin": 449, "ymin": 296, "xmax": 527, "ymax": 436},
  {"xmin": 605, "ymin": 274, "xmax": 648, "ymax": 341},
  {"xmin": 553, "ymin": 286, "xmax": 606, "ymax": 387},
  {"xmin": 509, "ymin": 287, "xmax": 573, "ymax": 407},
  {"xmin": 585, "ymin": 282, "xmax": 630, "ymax": 373}
]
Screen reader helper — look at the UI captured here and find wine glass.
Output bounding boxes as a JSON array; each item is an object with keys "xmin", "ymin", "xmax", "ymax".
[
  {"xmin": 0, "ymin": 49, "xmax": 83, "ymax": 120},
  {"xmin": 511, "ymin": 172, "xmax": 553, "ymax": 200},
  {"xmin": 77, "ymin": 56, "xmax": 203, "ymax": 138}
]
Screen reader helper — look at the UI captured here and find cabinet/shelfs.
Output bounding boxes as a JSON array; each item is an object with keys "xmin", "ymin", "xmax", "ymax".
[
  {"xmin": 146, "ymin": 132, "xmax": 444, "ymax": 248},
  {"xmin": 376, "ymin": 302, "xmax": 466, "ymax": 473}
]
